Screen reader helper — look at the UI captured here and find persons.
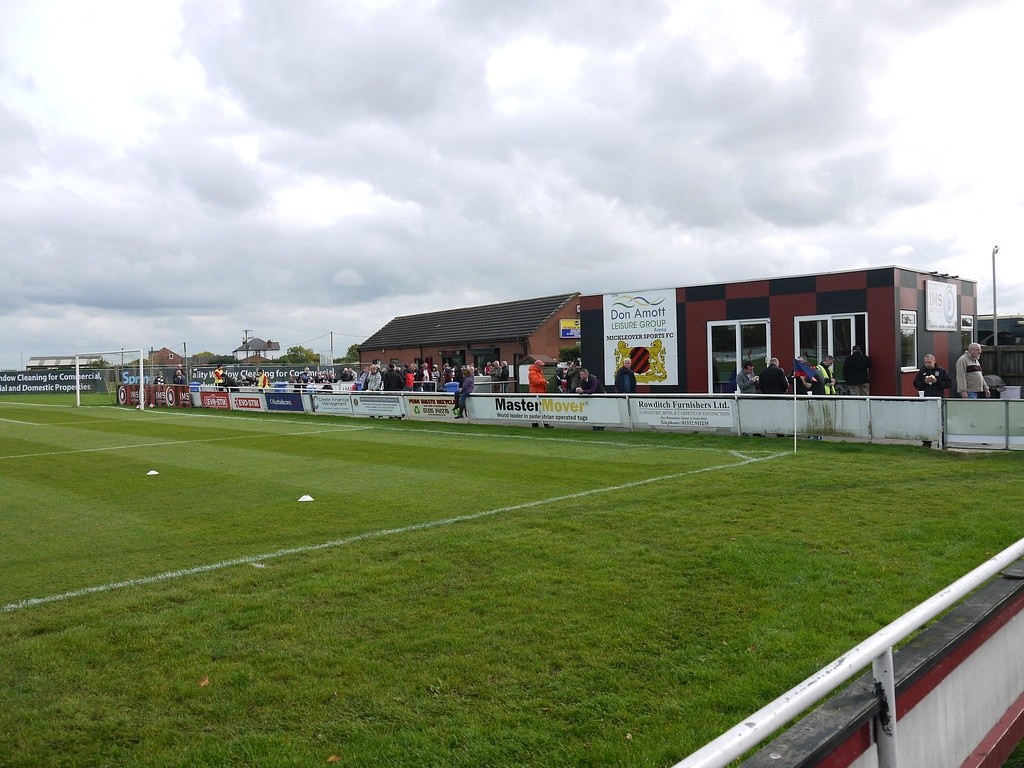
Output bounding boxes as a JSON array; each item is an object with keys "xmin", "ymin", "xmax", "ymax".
[
  {"xmin": 955, "ymin": 343, "xmax": 991, "ymax": 398},
  {"xmin": 736, "ymin": 356, "xmax": 837, "ymax": 440},
  {"xmin": 173, "ymin": 364, "xmax": 186, "ymax": 384},
  {"xmin": 527, "ymin": 360, "xmax": 554, "ymax": 428},
  {"xmin": 576, "ymin": 369, "xmax": 606, "ymax": 430},
  {"xmin": 559, "ymin": 359, "xmax": 584, "ymax": 393},
  {"xmin": 843, "ymin": 346, "xmax": 871, "ymax": 396},
  {"xmin": 290, "ymin": 360, "xmax": 509, "ymax": 419},
  {"xmin": 154, "ymin": 371, "xmax": 167, "ymax": 386},
  {"xmin": 250, "ymin": 369, "xmax": 269, "ymax": 392},
  {"xmin": 913, "ymin": 354, "xmax": 951, "ymax": 448},
  {"xmin": 214, "ymin": 364, "xmax": 239, "ymax": 392},
  {"xmin": 615, "ymin": 358, "xmax": 636, "ymax": 394}
]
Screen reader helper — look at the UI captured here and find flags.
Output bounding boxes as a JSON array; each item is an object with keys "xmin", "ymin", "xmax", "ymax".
[{"xmin": 794, "ymin": 359, "xmax": 815, "ymax": 382}]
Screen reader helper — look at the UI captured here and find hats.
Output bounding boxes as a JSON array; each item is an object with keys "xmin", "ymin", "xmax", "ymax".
[
  {"xmin": 851, "ymin": 346, "xmax": 860, "ymax": 351},
  {"xmin": 421, "ymin": 363, "xmax": 428, "ymax": 368},
  {"xmin": 824, "ymin": 355, "xmax": 835, "ymax": 361},
  {"xmin": 534, "ymin": 360, "xmax": 545, "ymax": 366},
  {"xmin": 433, "ymin": 364, "xmax": 438, "ymax": 370},
  {"xmin": 444, "ymin": 363, "xmax": 449, "ymax": 367}
]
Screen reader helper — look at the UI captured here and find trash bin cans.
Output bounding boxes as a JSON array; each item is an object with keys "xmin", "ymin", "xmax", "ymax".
[
  {"xmin": 444, "ymin": 382, "xmax": 459, "ymax": 393},
  {"xmin": 413, "ymin": 381, "xmax": 437, "ymax": 393},
  {"xmin": 275, "ymin": 381, "xmax": 288, "ymax": 393},
  {"xmin": 189, "ymin": 381, "xmax": 201, "ymax": 393},
  {"xmin": 977, "ymin": 375, "xmax": 1005, "ymax": 399}
]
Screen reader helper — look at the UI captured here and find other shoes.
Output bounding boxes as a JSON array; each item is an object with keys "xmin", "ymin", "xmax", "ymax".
[
  {"xmin": 454, "ymin": 413, "xmax": 463, "ymax": 419},
  {"xmin": 452, "ymin": 405, "xmax": 460, "ymax": 409},
  {"xmin": 753, "ymin": 433, "xmax": 766, "ymax": 437},
  {"xmin": 776, "ymin": 434, "xmax": 784, "ymax": 438},
  {"xmin": 787, "ymin": 434, "xmax": 798, "ymax": 438},
  {"xmin": 531, "ymin": 423, "xmax": 539, "ymax": 427},
  {"xmin": 593, "ymin": 427, "xmax": 604, "ymax": 431},
  {"xmin": 809, "ymin": 435, "xmax": 823, "ymax": 440},
  {"xmin": 544, "ymin": 424, "xmax": 554, "ymax": 428}
]
